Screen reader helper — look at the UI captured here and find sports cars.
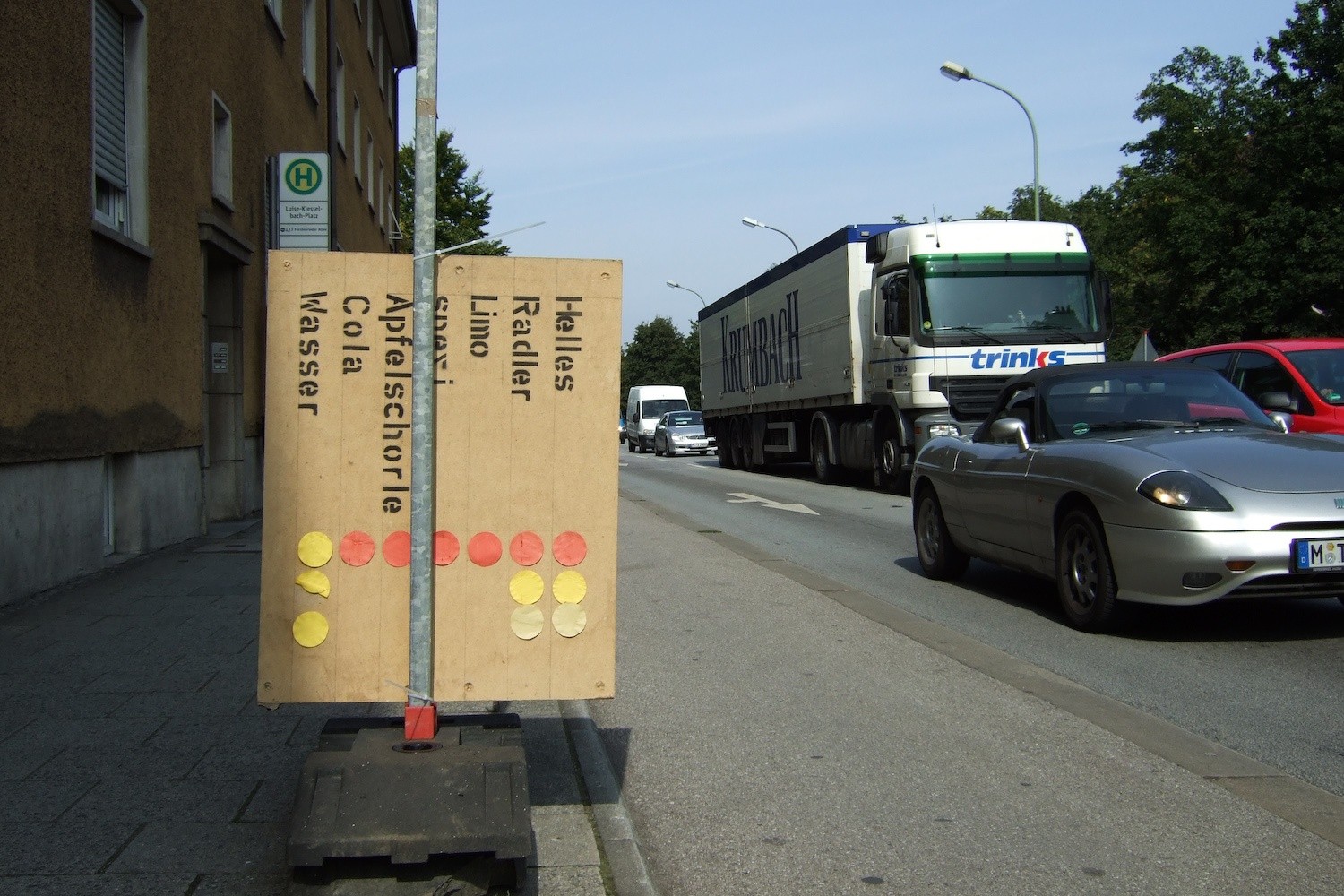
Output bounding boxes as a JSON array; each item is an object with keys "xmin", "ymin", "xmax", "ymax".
[{"xmin": 905, "ymin": 360, "xmax": 1343, "ymax": 631}]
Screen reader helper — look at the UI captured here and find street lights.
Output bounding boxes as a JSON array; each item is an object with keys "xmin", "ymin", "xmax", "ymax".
[{"xmin": 937, "ymin": 59, "xmax": 1043, "ymax": 221}]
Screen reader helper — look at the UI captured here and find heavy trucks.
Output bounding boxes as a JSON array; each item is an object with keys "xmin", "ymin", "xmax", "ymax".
[{"xmin": 698, "ymin": 219, "xmax": 1121, "ymax": 495}]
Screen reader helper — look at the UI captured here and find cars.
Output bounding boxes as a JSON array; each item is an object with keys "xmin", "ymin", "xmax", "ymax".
[
  {"xmin": 1131, "ymin": 335, "xmax": 1344, "ymax": 437},
  {"xmin": 652, "ymin": 411, "xmax": 719, "ymax": 456}
]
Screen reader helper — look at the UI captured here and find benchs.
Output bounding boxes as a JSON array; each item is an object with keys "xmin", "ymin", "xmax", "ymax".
[{"xmin": 1009, "ymin": 393, "xmax": 1181, "ymax": 444}]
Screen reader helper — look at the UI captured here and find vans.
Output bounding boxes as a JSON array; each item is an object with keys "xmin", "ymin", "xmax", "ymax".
[{"xmin": 625, "ymin": 385, "xmax": 691, "ymax": 454}]
